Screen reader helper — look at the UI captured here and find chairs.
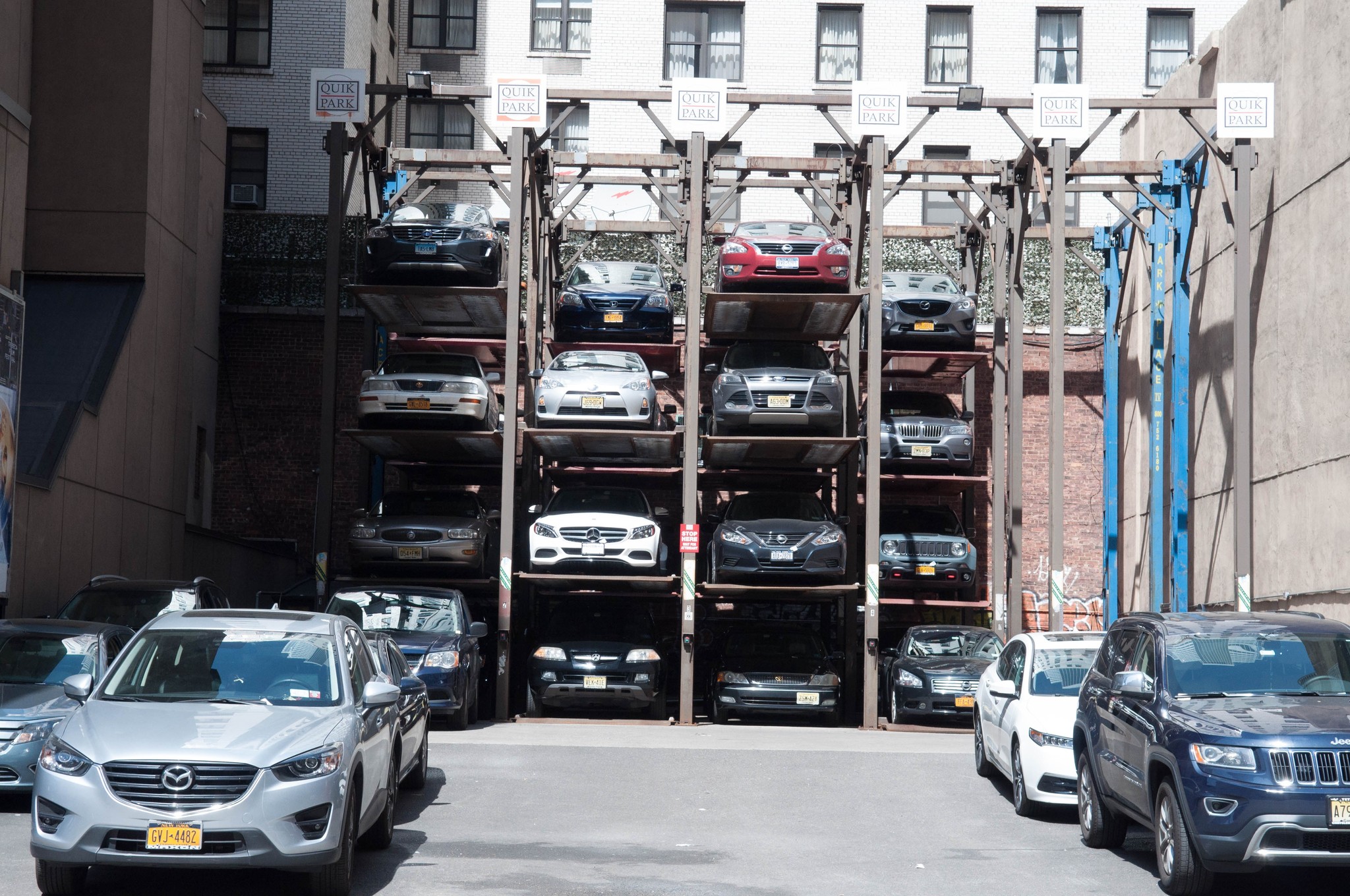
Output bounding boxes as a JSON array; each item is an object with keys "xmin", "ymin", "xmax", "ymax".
[{"xmin": 265, "ymin": 653, "xmax": 322, "ymax": 696}]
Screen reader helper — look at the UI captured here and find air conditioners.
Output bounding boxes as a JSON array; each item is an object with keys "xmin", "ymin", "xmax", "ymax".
[{"xmin": 230, "ymin": 181, "xmax": 260, "ymax": 209}]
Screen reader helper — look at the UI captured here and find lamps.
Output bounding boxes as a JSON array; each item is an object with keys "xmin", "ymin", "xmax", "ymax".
[
  {"xmin": 957, "ymin": 81, "xmax": 984, "ymax": 112},
  {"xmin": 405, "ymin": 69, "xmax": 433, "ymax": 100}
]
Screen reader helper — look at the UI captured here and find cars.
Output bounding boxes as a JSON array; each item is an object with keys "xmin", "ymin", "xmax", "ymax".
[
  {"xmin": 973, "ymin": 631, "xmax": 1115, "ymax": 815},
  {"xmin": 706, "ymin": 489, "xmax": 852, "ymax": 584},
  {"xmin": 857, "ymin": 387, "xmax": 975, "ymax": 474},
  {"xmin": 518, "ymin": 602, "xmax": 678, "ymax": 715},
  {"xmin": 527, "ymin": 483, "xmax": 670, "ymax": 574},
  {"xmin": 28, "ymin": 601, "xmax": 399, "ymax": 895},
  {"xmin": 362, "ymin": 631, "xmax": 430, "ymax": 797},
  {"xmin": 706, "ymin": 622, "xmax": 846, "ymax": 724},
  {"xmin": 320, "ymin": 584, "xmax": 489, "ymax": 728},
  {"xmin": 707, "ymin": 220, "xmax": 853, "ymax": 293},
  {"xmin": 347, "ymin": 489, "xmax": 499, "ymax": 571},
  {"xmin": 359, "ymin": 351, "xmax": 503, "ymax": 430},
  {"xmin": 703, "ymin": 342, "xmax": 850, "ymax": 436},
  {"xmin": 0, "ymin": 618, "xmax": 137, "ymax": 798},
  {"xmin": 863, "ymin": 271, "xmax": 981, "ymax": 351},
  {"xmin": 877, "ymin": 504, "xmax": 982, "ymax": 597},
  {"xmin": 548, "ymin": 258, "xmax": 682, "ymax": 342},
  {"xmin": 528, "ymin": 351, "xmax": 669, "ymax": 429},
  {"xmin": 880, "ymin": 625, "xmax": 1012, "ymax": 723},
  {"xmin": 364, "ymin": 201, "xmax": 510, "ymax": 284}
]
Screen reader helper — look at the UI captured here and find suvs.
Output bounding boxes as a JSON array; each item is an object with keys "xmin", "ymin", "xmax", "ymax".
[
  {"xmin": 58, "ymin": 575, "xmax": 230, "ymax": 638},
  {"xmin": 1072, "ymin": 610, "xmax": 1350, "ymax": 896}
]
